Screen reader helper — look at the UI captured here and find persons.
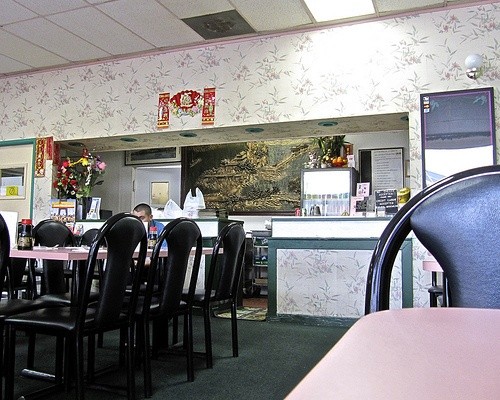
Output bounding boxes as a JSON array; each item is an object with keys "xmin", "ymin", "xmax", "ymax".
[{"xmin": 132, "ymin": 203, "xmax": 167, "ymax": 287}]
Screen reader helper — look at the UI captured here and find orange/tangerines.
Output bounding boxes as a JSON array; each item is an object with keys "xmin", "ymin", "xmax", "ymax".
[{"xmin": 332, "ymin": 157, "xmax": 347, "ymax": 164}]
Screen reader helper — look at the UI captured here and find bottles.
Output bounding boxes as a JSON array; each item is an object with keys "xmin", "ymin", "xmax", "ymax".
[
  {"xmin": 148, "ymin": 227, "xmax": 158, "ymax": 250},
  {"xmin": 303, "ymin": 145, "xmax": 351, "ymax": 169},
  {"xmin": 16, "ymin": 218, "xmax": 34, "ymax": 250},
  {"xmin": 295, "ymin": 204, "xmax": 320, "ymax": 217}
]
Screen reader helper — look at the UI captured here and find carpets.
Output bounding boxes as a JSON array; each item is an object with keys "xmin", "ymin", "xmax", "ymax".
[{"xmin": 217, "ymin": 304, "xmax": 268, "ymax": 321}]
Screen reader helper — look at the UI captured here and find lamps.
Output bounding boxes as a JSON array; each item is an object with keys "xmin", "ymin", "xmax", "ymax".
[{"xmin": 464, "ymin": 53, "xmax": 483, "ymax": 80}]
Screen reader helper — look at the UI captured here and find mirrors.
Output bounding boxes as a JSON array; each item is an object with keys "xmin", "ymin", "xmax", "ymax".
[{"xmin": 420, "ymin": 86, "xmax": 497, "ymax": 188}]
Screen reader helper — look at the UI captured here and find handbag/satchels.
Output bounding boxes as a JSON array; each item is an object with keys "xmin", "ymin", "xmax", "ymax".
[
  {"xmin": 183, "ymin": 187, "xmax": 206, "ymax": 210},
  {"xmin": 162, "ymin": 199, "xmax": 183, "ymax": 219}
]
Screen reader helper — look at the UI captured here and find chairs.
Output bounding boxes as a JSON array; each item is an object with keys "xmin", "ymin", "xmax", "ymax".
[
  {"xmin": 364, "ymin": 164, "xmax": 500, "ymax": 315},
  {"xmin": 0, "ymin": 213, "xmax": 248, "ymax": 400}
]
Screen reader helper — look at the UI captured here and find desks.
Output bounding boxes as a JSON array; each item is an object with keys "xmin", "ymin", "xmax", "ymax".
[
  {"xmin": 9, "ymin": 243, "xmax": 224, "ymax": 395},
  {"xmin": 282, "ymin": 305, "xmax": 500, "ymax": 400}
]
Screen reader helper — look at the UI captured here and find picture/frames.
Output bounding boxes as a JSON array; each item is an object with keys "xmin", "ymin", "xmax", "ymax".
[
  {"xmin": 124, "ymin": 146, "xmax": 182, "ymax": 165},
  {"xmin": 180, "ymin": 136, "xmax": 341, "ymax": 216}
]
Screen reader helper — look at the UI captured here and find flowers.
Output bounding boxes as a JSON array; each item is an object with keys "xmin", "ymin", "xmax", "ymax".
[{"xmin": 52, "ymin": 149, "xmax": 107, "ymax": 197}]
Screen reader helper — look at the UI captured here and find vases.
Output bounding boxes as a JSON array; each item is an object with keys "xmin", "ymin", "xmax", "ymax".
[{"xmin": 81, "ymin": 195, "xmax": 92, "ymax": 220}]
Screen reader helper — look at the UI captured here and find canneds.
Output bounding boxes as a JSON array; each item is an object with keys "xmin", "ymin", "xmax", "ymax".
[
  {"xmin": 296, "ymin": 209, "xmax": 301, "ymax": 216},
  {"xmin": 302, "ymin": 208, "xmax": 306, "ymax": 216}
]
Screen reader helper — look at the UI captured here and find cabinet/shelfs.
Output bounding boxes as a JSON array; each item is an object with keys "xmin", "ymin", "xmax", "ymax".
[{"xmin": 250, "ymin": 233, "xmax": 268, "ymax": 297}]
